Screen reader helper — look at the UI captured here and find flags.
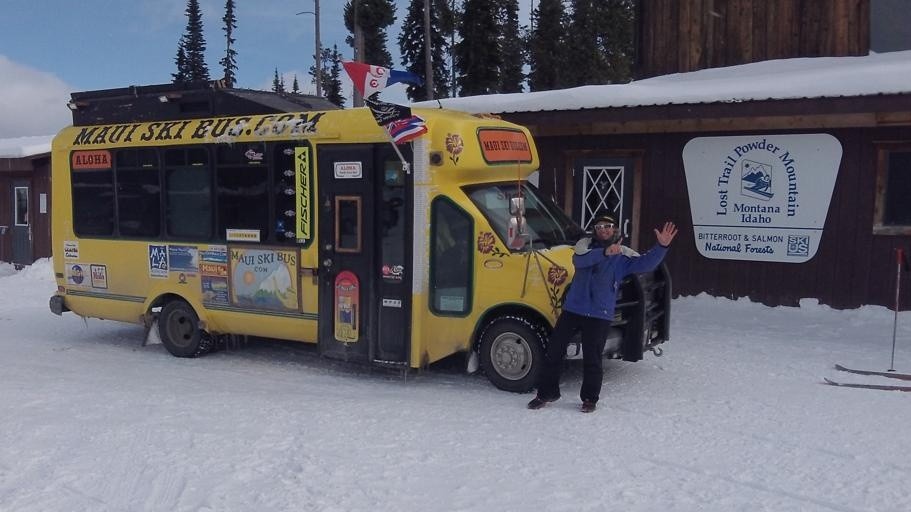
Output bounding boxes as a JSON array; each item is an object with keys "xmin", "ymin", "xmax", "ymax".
[
  {"xmin": 368, "ymin": 101, "xmax": 413, "ymax": 124},
  {"xmin": 345, "ymin": 63, "xmax": 428, "ymax": 103},
  {"xmin": 382, "ymin": 113, "xmax": 430, "ymax": 147}
]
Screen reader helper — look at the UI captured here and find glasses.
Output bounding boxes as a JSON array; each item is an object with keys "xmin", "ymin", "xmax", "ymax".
[{"xmin": 594, "ymin": 223, "xmax": 615, "ymax": 233}]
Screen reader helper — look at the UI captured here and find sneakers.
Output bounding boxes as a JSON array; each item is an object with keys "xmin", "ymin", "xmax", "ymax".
[
  {"xmin": 527, "ymin": 391, "xmax": 561, "ymax": 409},
  {"xmin": 579, "ymin": 398, "xmax": 597, "ymax": 412}
]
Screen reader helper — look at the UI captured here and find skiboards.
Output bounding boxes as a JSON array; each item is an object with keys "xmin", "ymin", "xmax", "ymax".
[{"xmin": 824, "ymin": 364, "xmax": 911, "ymax": 391}]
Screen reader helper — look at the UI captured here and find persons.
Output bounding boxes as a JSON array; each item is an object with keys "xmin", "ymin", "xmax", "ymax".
[{"xmin": 521, "ymin": 208, "xmax": 682, "ymax": 411}]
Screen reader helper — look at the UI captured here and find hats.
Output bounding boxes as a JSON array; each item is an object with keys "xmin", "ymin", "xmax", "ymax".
[{"xmin": 593, "ymin": 208, "xmax": 619, "ymax": 228}]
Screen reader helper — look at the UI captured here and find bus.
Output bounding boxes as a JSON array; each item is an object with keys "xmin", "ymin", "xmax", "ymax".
[{"xmin": 49, "ymin": 106, "xmax": 672, "ymax": 393}]
